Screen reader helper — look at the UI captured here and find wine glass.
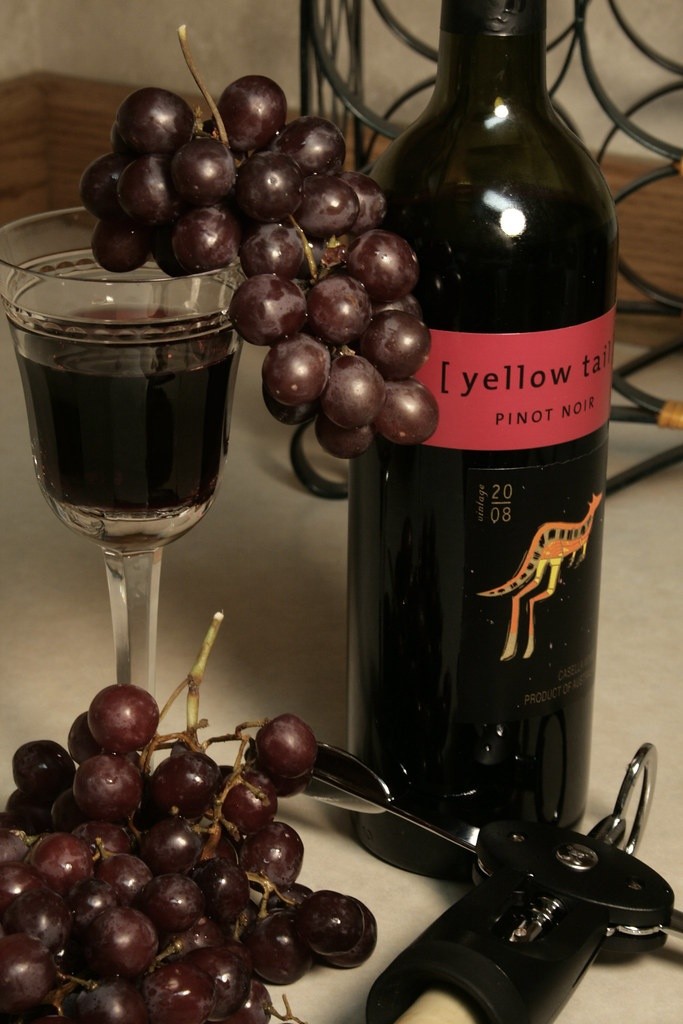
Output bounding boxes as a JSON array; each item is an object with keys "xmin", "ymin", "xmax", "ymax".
[{"xmin": 0, "ymin": 206, "xmax": 240, "ymax": 696}]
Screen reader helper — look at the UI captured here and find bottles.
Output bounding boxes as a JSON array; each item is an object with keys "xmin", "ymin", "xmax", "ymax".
[{"xmin": 344, "ymin": 0, "xmax": 619, "ymax": 882}]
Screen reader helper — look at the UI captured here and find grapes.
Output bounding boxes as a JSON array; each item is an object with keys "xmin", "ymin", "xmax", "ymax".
[
  {"xmin": 79, "ymin": 72, "xmax": 438, "ymax": 459},
  {"xmin": 0, "ymin": 682, "xmax": 377, "ymax": 1024}
]
